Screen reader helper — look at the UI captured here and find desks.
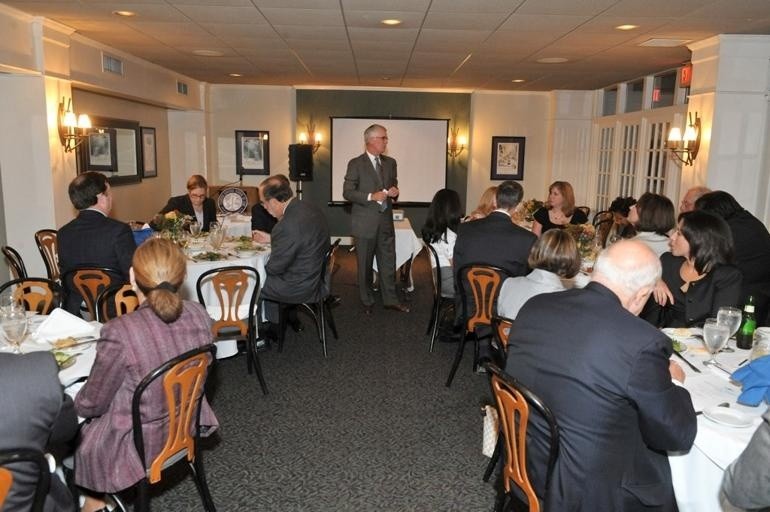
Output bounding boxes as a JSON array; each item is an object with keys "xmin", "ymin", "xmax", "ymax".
[
  {"xmin": 332, "ymin": 217, "xmax": 413, "ymax": 302},
  {"xmin": 660, "ymin": 326, "xmax": 770, "ymax": 511}
]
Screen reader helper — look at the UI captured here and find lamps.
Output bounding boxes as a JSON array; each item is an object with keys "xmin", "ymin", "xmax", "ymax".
[
  {"xmin": 61, "ymin": 98, "xmax": 92, "ymax": 152},
  {"xmin": 300, "ymin": 124, "xmax": 322, "ymax": 154},
  {"xmin": 665, "ymin": 111, "xmax": 697, "ymax": 166},
  {"xmin": 446, "ymin": 127, "xmax": 466, "ymax": 158}
]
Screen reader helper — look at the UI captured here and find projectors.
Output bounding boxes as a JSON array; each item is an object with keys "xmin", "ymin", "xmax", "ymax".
[{"xmin": 392, "ymin": 209, "xmax": 405, "ymax": 221}]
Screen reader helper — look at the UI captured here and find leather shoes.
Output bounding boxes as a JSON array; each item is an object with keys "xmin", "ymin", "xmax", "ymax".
[
  {"xmin": 362, "ymin": 304, "xmax": 374, "ymax": 317},
  {"xmin": 328, "ymin": 295, "xmax": 342, "ymax": 309},
  {"xmin": 238, "ymin": 338, "xmax": 270, "ymax": 353},
  {"xmin": 291, "ymin": 311, "xmax": 306, "ymax": 332},
  {"xmin": 384, "ymin": 302, "xmax": 410, "ymax": 313}
]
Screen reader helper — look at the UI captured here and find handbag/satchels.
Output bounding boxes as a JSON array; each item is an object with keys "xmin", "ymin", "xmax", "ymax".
[{"xmin": 479, "ymin": 400, "xmax": 502, "ymax": 459}]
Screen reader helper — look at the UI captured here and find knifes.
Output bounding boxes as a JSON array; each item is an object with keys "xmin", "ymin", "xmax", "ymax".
[
  {"xmin": 671, "ymin": 347, "xmax": 701, "ymax": 374},
  {"xmin": 59, "ymin": 352, "xmax": 84, "ymax": 366},
  {"xmin": 228, "ymin": 253, "xmax": 240, "ymax": 259}
]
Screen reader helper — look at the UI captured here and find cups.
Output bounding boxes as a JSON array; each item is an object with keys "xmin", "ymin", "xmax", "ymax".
[
  {"xmin": 209, "ymin": 222, "xmax": 223, "ymax": 250},
  {"xmin": 750, "ymin": 326, "xmax": 770, "ymax": 362},
  {"xmin": 1, "ymin": 291, "xmax": 24, "ymax": 312},
  {"xmin": 609, "ymin": 234, "xmax": 623, "ymax": 246}
]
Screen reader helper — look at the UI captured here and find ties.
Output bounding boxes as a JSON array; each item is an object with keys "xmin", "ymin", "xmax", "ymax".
[{"xmin": 375, "ymin": 156, "xmax": 388, "ymax": 214}]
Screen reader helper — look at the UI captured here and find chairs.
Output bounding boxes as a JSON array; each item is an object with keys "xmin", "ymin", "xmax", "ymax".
[
  {"xmin": 196, "ymin": 226, "xmax": 342, "ymax": 405},
  {"xmin": 422, "ymin": 206, "xmax": 617, "ymax": 508},
  {"xmin": 0, "ymin": 226, "xmax": 215, "ymax": 512}
]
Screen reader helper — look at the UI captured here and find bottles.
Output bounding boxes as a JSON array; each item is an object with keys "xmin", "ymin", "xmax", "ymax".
[{"xmin": 735, "ymin": 294, "xmax": 756, "ymax": 350}]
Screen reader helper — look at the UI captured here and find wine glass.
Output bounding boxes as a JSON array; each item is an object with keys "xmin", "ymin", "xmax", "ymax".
[
  {"xmin": 716, "ymin": 304, "xmax": 743, "ymax": 353},
  {"xmin": 177, "ymin": 231, "xmax": 189, "ymax": 253},
  {"xmin": 702, "ymin": 317, "xmax": 730, "ymax": 370},
  {"xmin": 189, "ymin": 221, "xmax": 202, "ymax": 245},
  {"xmin": 0, "ymin": 311, "xmax": 29, "ymax": 355}
]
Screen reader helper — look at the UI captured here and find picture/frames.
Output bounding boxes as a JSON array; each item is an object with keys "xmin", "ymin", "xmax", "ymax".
[
  {"xmin": 140, "ymin": 126, "xmax": 157, "ymax": 179},
  {"xmin": 76, "ymin": 113, "xmax": 142, "ymax": 186},
  {"xmin": 235, "ymin": 129, "xmax": 270, "ymax": 175},
  {"xmin": 490, "ymin": 136, "xmax": 525, "ymax": 180}
]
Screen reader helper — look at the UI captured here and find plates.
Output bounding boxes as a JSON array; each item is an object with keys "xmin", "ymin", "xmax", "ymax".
[
  {"xmin": 703, "ymin": 401, "xmax": 753, "ymax": 429},
  {"xmin": 217, "ymin": 188, "xmax": 248, "ymax": 215},
  {"xmin": 235, "ymin": 249, "xmax": 259, "ymax": 255},
  {"xmin": 669, "ymin": 339, "xmax": 689, "ymax": 353},
  {"xmin": 59, "ymin": 337, "xmax": 94, "ymax": 355},
  {"xmin": 578, "ymin": 262, "xmax": 594, "ymax": 277},
  {"xmin": 56, "ymin": 353, "xmax": 75, "ymax": 373},
  {"xmin": 661, "ymin": 325, "xmax": 695, "ymax": 340},
  {"xmin": 189, "ymin": 251, "xmax": 228, "ymax": 262}
]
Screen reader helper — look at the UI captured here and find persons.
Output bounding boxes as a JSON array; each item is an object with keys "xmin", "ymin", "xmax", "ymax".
[
  {"xmin": 341, "ymin": 124, "xmax": 410, "ymax": 317},
  {"xmin": 504, "ymin": 236, "xmax": 696, "ymax": 512},
  {"xmin": 720, "ymin": 405, "xmax": 769, "ymax": 512},
  {"xmin": 56, "ymin": 170, "xmax": 136, "ymax": 322},
  {"xmin": 2, "ymin": 352, "xmax": 83, "ymax": 512},
  {"xmin": 66, "ymin": 236, "xmax": 220, "ymax": 512},
  {"xmin": 424, "ymin": 178, "xmax": 770, "ymax": 348},
  {"xmin": 151, "ymin": 176, "xmax": 217, "ymax": 233},
  {"xmin": 254, "ymin": 177, "xmax": 332, "ymax": 347}
]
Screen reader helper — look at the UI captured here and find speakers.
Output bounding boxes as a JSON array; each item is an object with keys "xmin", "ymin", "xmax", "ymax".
[{"xmin": 288, "ymin": 143, "xmax": 313, "ymax": 181}]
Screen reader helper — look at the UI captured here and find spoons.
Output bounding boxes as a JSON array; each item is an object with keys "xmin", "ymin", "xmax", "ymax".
[{"xmin": 695, "ymin": 402, "xmax": 731, "ymax": 416}]
